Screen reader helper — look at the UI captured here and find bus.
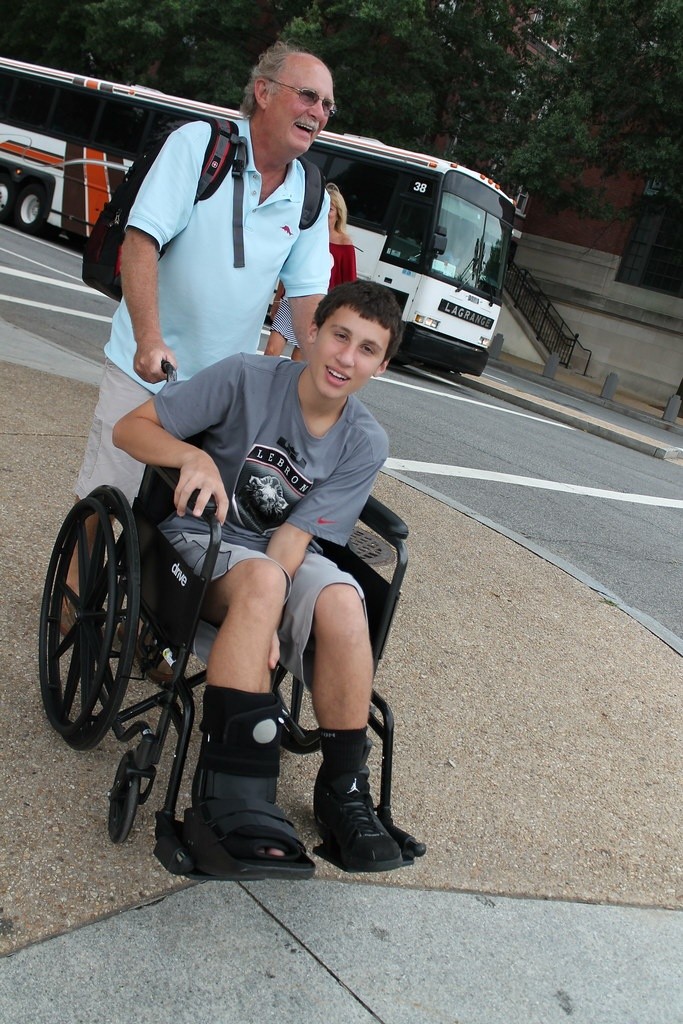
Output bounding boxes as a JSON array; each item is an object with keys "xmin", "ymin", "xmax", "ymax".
[{"xmin": 0, "ymin": 57, "xmax": 517, "ymax": 377}]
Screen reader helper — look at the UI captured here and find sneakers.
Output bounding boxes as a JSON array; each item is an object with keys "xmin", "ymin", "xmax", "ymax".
[{"xmin": 312, "ymin": 764, "xmax": 403, "ymax": 872}]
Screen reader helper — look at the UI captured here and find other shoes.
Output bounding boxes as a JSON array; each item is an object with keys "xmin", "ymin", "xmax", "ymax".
[
  {"xmin": 61, "ymin": 595, "xmax": 77, "ymax": 635},
  {"xmin": 118, "ymin": 617, "xmax": 177, "ymax": 686}
]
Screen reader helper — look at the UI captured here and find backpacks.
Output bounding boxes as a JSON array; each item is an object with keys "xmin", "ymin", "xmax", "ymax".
[{"xmin": 82, "ymin": 118, "xmax": 326, "ymax": 304}]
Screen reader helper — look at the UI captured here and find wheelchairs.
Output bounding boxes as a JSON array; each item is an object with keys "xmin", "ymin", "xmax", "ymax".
[{"xmin": 39, "ymin": 358, "xmax": 426, "ymax": 883}]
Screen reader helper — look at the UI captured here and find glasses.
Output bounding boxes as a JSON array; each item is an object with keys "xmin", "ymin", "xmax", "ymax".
[{"xmin": 264, "ymin": 76, "xmax": 339, "ymax": 117}]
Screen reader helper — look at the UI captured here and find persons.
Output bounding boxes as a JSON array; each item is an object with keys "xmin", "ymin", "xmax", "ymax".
[
  {"xmin": 56, "ymin": 40, "xmax": 336, "ymax": 687},
  {"xmin": 263, "ymin": 183, "xmax": 357, "ymax": 363},
  {"xmin": 114, "ymin": 279, "xmax": 405, "ymax": 870}
]
